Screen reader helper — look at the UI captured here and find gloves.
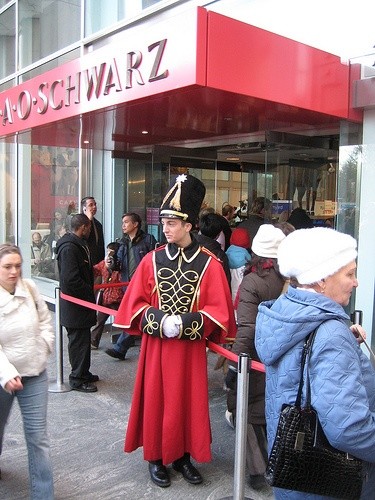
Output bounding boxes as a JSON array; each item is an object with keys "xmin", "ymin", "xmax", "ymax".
[{"xmin": 162, "ymin": 313, "xmax": 182, "ymax": 338}]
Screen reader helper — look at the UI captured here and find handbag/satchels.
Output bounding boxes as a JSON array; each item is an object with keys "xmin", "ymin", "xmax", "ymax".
[
  {"xmin": 38, "ymin": 261, "xmax": 52, "ymax": 273},
  {"xmin": 263, "ymin": 318, "xmax": 364, "ymax": 500}
]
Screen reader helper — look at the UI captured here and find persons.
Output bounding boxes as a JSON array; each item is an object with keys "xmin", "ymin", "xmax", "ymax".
[
  {"xmin": 0, "ymin": 243, "xmax": 54, "ymax": 500},
  {"xmin": 112, "ymin": 217, "xmax": 237, "ymax": 488},
  {"xmin": 255, "ymin": 227, "xmax": 375, "ymax": 500},
  {"xmin": 31, "ymin": 233, "xmax": 52, "ymax": 278},
  {"xmin": 106, "ymin": 213, "xmax": 159, "ymax": 360},
  {"xmin": 81, "ymin": 197, "xmax": 105, "ymax": 327},
  {"xmin": 50, "ymin": 209, "xmax": 70, "ymax": 241},
  {"xmin": 91, "ymin": 242, "xmax": 136, "ymax": 350},
  {"xmin": 197, "ymin": 198, "xmax": 330, "ymax": 491},
  {"xmin": 55, "ymin": 214, "xmax": 99, "ymax": 392}
]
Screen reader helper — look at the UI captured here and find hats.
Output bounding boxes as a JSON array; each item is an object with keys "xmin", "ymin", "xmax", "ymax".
[
  {"xmin": 156, "ymin": 172, "xmax": 206, "ymax": 225},
  {"xmin": 229, "ymin": 227, "xmax": 251, "ymax": 249},
  {"xmin": 277, "ymin": 226, "xmax": 357, "ymax": 284},
  {"xmin": 251, "ymin": 223, "xmax": 286, "ymax": 259},
  {"xmin": 288, "ymin": 207, "xmax": 312, "ymax": 228}
]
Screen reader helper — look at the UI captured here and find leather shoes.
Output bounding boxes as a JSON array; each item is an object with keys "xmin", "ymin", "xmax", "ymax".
[
  {"xmin": 148, "ymin": 459, "xmax": 171, "ymax": 488},
  {"xmin": 172, "ymin": 458, "xmax": 204, "ymax": 484}
]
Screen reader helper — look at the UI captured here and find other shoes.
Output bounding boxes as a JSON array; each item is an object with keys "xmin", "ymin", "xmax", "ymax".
[
  {"xmin": 71, "ymin": 381, "xmax": 97, "ymax": 392},
  {"xmin": 225, "ymin": 409, "xmax": 235, "ymax": 429},
  {"xmin": 91, "ymin": 332, "xmax": 99, "ymax": 348},
  {"xmin": 106, "ymin": 348, "xmax": 126, "ymax": 360},
  {"xmin": 89, "ymin": 375, "xmax": 99, "ymax": 383},
  {"xmin": 250, "ymin": 473, "xmax": 266, "ymax": 490}
]
